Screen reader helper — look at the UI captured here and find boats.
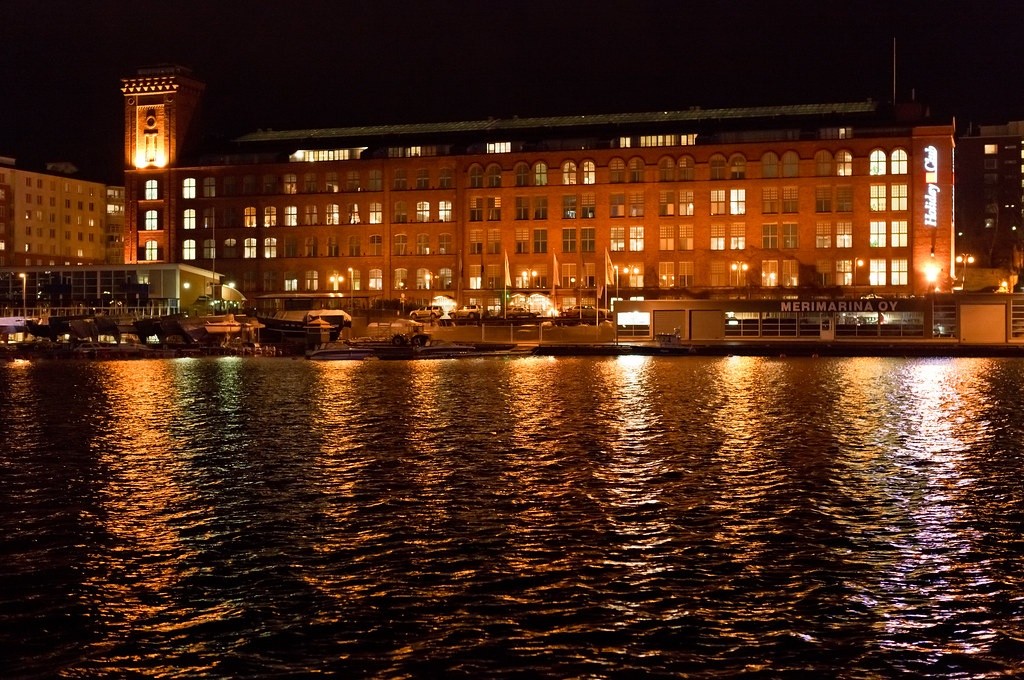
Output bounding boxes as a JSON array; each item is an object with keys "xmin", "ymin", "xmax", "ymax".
[
  {"xmin": 305, "ymin": 342, "xmax": 374, "ymax": 360},
  {"xmin": 204, "ymin": 321, "xmax": 265, "ymax": 333},
  {"xmin": 257, "ymin": 309, "xmax": 352, "ymax": 349}
]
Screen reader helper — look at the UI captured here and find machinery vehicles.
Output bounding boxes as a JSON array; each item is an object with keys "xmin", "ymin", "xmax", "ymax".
[{"xmin": 392, "ymin": 325, "xmax": 432, "ymax": 346}]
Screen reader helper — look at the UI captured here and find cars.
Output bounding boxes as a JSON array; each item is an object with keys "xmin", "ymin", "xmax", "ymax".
[
  {"xmin": 449, "ymin": 305, "xmax": 483, "ymax": 319},
  {"xmin": 506, "ymin": 307, "xmax": 540, "ymax": 319},
  {"xmin": 560, "ymin": 305, "xmax": 610, "ymax": 318},
  {"xmin": 410, "ymin": 305, "xmax": 444, "ymax": 319}
]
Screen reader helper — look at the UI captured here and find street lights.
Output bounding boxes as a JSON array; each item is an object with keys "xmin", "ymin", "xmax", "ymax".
[
  {"xmin": 348, "ymin": 268, "xmax": 354, "ymax": 317},
  {"xmin": 614, "ymin": 265, "xmax": 619, "ymax": 300},
  {"xmin": 732, "ymin": 261, "xmax": 749, "ymax": 286},
  {"xmin": 955, "ymin": 253, "xmax": 974, "ymax": 291},
  {"xmin": 854, "ymin": 257, "xmax": 864, "ymax": 297},
  {"xmin": 20, "ymin": 273, "xmax": 26, "ymax": 325}
]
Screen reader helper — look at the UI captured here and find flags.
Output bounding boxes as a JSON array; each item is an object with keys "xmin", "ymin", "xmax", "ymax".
[
  {"xmin": 604, "ymin": 246, "xmax": 615, "ymax": 286},
  {"xmin": 457, "ymin": 249, "xmax": 463, "ymax": 288},
  {"xmin": 504, "ymin": 248, "xmax": 512, "ymax": 287},
  {"xmin": 552, "ymin": 248, "xmax": 561, "ymax": 287}
]
